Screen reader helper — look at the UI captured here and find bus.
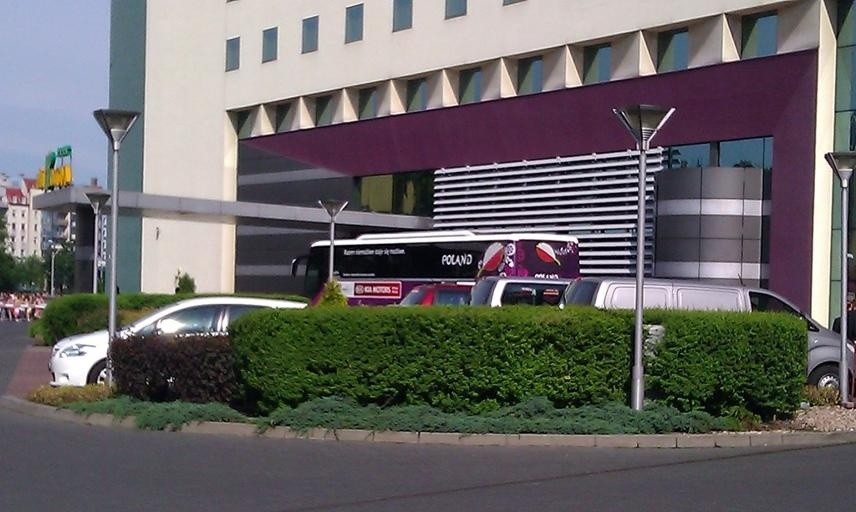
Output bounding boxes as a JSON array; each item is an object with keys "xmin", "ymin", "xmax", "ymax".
[{"xmin": 289, "ymin": 228, "xmax": 583, "ymax": 313}]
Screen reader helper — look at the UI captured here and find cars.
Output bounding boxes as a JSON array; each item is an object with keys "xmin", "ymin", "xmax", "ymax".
[
  {"xmin": 467, "ymin": 273, "xmax": 575, "ymax": 309},
  {"xmin": 47, "ymin": 294, "xmax": 312, "ymax": 391},
  {"xmin": 399, "ymin": 282, "xmax": 473, "ymax": 307}
]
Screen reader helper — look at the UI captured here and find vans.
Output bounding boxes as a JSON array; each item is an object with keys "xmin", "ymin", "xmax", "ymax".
[{"xmin": 555, "ymin": 274, "xmax": 855, "ymax": 407}]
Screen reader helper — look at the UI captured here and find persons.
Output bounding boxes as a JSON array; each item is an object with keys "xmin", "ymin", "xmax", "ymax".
[
  {"xmin": 831, "ymin": 299, "xmax": 856, "ymax": 342},
  {"xmin": 1, "ymin": 291, "xmax": 47, "ymax": 324}
]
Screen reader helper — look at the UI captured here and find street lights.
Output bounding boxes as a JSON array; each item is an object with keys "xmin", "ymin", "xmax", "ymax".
[
  {"xmin": 315, "ymin": 198, "xmax": 350, "ymax": 291},
  {"xmin": 83, "ymin": 190, "xmax": 111, "ymax": 296},
  {"xmin": 45, "ymin": 240, "xmax": 64, "ymax": 298},
  {"xmin": 823, "ymin": 149, "xmax": 855, "ymax": 409},
  {"xmin": 610, "ymin": 101, "xmax": 679, "ymax": 413},
  {"xmin": 89, "ymin": 106, "xmax": 145, "ymax": 391}
]
